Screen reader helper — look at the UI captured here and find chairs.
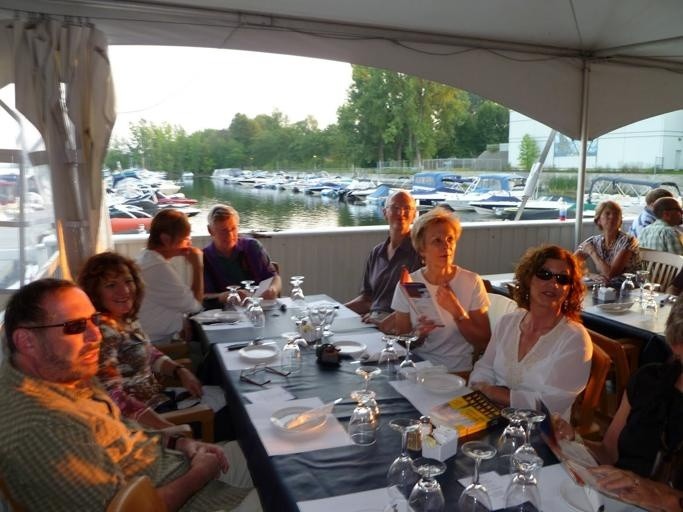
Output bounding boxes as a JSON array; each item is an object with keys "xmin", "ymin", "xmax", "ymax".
[
  {"xmin": 104, "ymin": 475, "xmax": 168, "ymax": 512},
  {"xmin": 568, "ymin": 341, "xmax": 615, "ymax": 443},
  {"xmin": 633, "ymin": 252, "xmax": 683, "ymax": 295},
  {"xmin": 483, "ymin": 280, "xmax": 494, "ymax": 291},
  {"xmin": 144, "ymin": 342, "xmax": 215, "ymax": 440},
  {"xmin": 580, "ymin": 326, "xmax": 637, "ymax": 424},
  {"xmin": 506, "ymin": 283, "xmax": 519, "ymax": 300}
]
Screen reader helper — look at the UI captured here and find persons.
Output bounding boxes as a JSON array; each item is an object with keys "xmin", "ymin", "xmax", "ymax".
[
  {"xmin": 79, "ymin": 251, "xmax": 228, "ymax": 437},
  {"xmin": 132, "ymin": 210, "xmax": 204, "ymax": 377},
  {"xmin": 468, "ymin": 245, "xmax": 593, "ymax": 424},
  {"xmin": 201, "ymin": 204, "xmax": 282, "ymax": 309},
  {"xmin": 391, "ymin": 208, "xmax": 491, "ymax": 381},
  {"xmin": 665, "ymin": 266, "xmax": 683, "ymax": 296},
  {"xmin": 554, "ymin": 293, "xmax": 683, "ymax": 512},
  {"xmin": 629, "ymin": 189, "xmax": 673, "ymax": 238},
  {"xmin": 343, "ymin": 192, "xmax": 425, "ymax": 325},
  {"xmin": 0, "ymin": 277, "xmax": 264, "ymax": 511},
  {"xmin": 639, "ymin": 197, "xmax": 683, "ymax": 255},
  {"xmin": 573, "ymin": 201, "xmax": 643, "ymax": 288}
]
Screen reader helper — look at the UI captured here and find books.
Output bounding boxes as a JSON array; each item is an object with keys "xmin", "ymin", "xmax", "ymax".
[
  {"xmin": 537, "ymin": 395, "xmax": 646, "ymax": 507},
  {"xmin": 400, "ymin": 264, "xmax": 445, "ymax": 328},
  {"xmin": 428, "ymin": 390, "xmax": 504, "ymax": 438}
]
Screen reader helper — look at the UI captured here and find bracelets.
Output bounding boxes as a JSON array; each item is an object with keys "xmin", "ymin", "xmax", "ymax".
[{"xmin": 453, "ymin": 312, "xmax": 467, "ymax": 323}]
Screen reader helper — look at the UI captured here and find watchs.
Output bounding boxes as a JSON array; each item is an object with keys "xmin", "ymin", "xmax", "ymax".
[{"xmin": 173, "ymin": 365, "xmax": 184, "ymax": 376}]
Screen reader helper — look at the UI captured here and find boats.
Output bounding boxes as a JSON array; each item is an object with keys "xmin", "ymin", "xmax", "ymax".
[
  {"xmin": 366, "ymin": 185, "xmax": 408, "ymax": 207},
  {"xmin": 131, "ymin": 203, "xmax": 201, "ymax": 219},
  {"xmin": 107, "ymin": 175, "xmax": 184, "ymax": 199},
  {"xmin": 410, "ymin": 174, "xmax": 528, "ymax": 216},
  {"xmin": 105, "ymin": 167, "xmax": 185, "ymax": 198},
  {"xmin": 382, "ymin": 170, "xmax": 466, "ymax": 208},
  {"xmin": 127, "ymin": 194, "xmax": 198, "ymax": 209},
  {"xmin": 100, "ymin": 207, "xmax": 153, "ymax": 235},
  {"xmin": 212, "ymin": 166, "xmax": 295, "ymax": 189},
  {"xmin": 210, "ymin": 168, "xmax": 349, "ymax": 196},
  {"xmin": 107, "ymin": 204, "xmax": 155, "ymax": 234},
  {"xmin": 368, "ymin": 183, "xmax": 399, "ymax": 205},
  {"xmin": 275, "ymin": 176, "xmax": 400, "ymax": 204},
  {"xmin": 134, "ymin": 194, "xmax": 198, "ymax": 204},
  {"xmin": 467, "ymin": 175, "xmax": 576, "ymax": 219},
  {"xmin": 339, "ymin": 180, "xmax": 380, "ymax": 204},
  {"xmin": 124, "ymin": 199, "xmax": 202, "ymax": 220},
  {"xmin": 410, "ymin": 172, "xmax": 476, "ymax": 212},
  {"xmin": 469, "ymin": 193, "xmax": 576, "ymax": 217}
]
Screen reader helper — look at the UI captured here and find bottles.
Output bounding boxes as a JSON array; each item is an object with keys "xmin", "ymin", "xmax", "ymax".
[
  {"xmin": 407, "ymin": 419, "xmax": 422, "ymax": 453},
  {"xmin": 419, "ymin": 415, "xmax": 433, "ymax": 442},
  {"xmin": 592, "ymin": 284, "xmax": 606, "ymax": 300}
]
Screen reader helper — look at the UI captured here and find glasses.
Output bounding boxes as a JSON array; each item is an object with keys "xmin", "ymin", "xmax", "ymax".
[
  {"xmin": 665, "ymin": 207, "xmax": 681, "ymax": 211},
  {"xmin": 385, "ymin": 204, "xmax": 415, "ymax": 211},
  {"xmin": 535, "ymin": 270, "xmax": 572, "ymax": 285},
  {"xmin": 20, "ymin": 312, "xmax": 102, "ymax": 335}
]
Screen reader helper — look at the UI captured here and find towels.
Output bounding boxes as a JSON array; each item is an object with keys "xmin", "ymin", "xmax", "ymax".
[
  {"xmin": 189, "ymin": 310, "xmax": 254, "ymax": 330},
  {"xmin": 242, "ymin": 385, "xmax": 296, "ymax": 403},
  {"xmin": 295, "ymin": 485, "xmax": 416, "ymax": 512},
  {"xmin": 457, "ymin": 471, "xmax": 529, "ymax": 511},
  {"xmin": 216, "ymin": 339, "xmax": 288, "ymax": 371},
  {"xmin": 630, "ymin": 288, "xmax": 663, "ymax": 300},
  {"xmin": 233, "ymin": 295, "xmax": 299, "ymax": 312},
  {"xmin": 502, "ymin": 463, "xmax": 646, "ymax": 512},
  {"xmin": 582, "ymin": 303, "xmax": 652, "ymax": 325},
  {"xmin": 305, "ymin": 300, "xmax": 361, "ymax": 319},
  {"xmin": 490, "ymin": 280, "xmax": 514, "ymax": 289},
  {"xmin": 395, "ymin": 360, "xmax": 434, "ymax": 379},
  {"xmin": 243, "ymin": 396, "xmax": 356, "ymax": 457},
  {"xmin": 328, "ymin": 328, "xmax": 407, "ymax": 362},
  {"xmin": 388, "ymin": 380, "xmax": 473, "ymax": 415}
]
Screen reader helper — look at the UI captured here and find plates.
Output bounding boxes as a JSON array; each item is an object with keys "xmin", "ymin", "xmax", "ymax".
[
  {"xmin": 595, "ymin": 301, "xmax": 636, "ymax": 314},
  {"xmin": 581, "ymin": 281, "xmax": 603, "ymax": 288},
  {"xmin": 213, "ymin": 310, "xmax": 246, "ymax": 322},
  {"xmin": 269, "ymin": 405, "xmax": 330, "ymax": 433},
  {"xmin": 329, "ymin": 339, "xmax": 367, "ymax": 355},
  {"xmin": 417, "ymin": 371, "xmax": 466, "ymax": 393},
  {"xmin": 237, "ymin": 344, "xmax": 279, "ymax": 361},
  {"xmin": 558, "ymin": 475, "xmax": 592, "ymax": 511}
]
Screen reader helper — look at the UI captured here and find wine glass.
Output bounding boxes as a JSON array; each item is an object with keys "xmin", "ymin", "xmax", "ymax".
[
  {"xmin": 494, "ymin": 407, "xmax": 526, "ymax": 459},
  {"xmin": 640, "ymin": 281, "xmax": 661, "ymax": 324},
  {"xmin": 407, "ymin": 456, "xmax": 448, "ymax": 512},
  {"xmin": 306, "ymin": 306, "xmax": 323, "ymax": 343},
  {"xmin": 249, "ymin": 299, "xmax": 266, "ymax": 328},
  {"xmin": 455, "ymin": 440, "xmax": 498, "ymax": 512},
  {"xmin": 386, "ymin": 416, "xmax": 420, "ymax": 487},
  {"xmin": 378, "ymin": 334, "xmax": 401, "ymax": 373},
  {"xmin": 514, "ymin": 406, "xmax": 547, "ymax": 472},
  {"xmin": 354, "ymin": 364, "xmax": 383, "ymax": 417},
  {"xmin": 281, "ymin": 332, "xmax": 302, "ymax": 375},
  {"xmin": 396, "ymin": 334, "xmax": 419, "ymax": 382},
  {"xmin": 240, "ymin": 280, "xmax": 255, "ymax": 292},
  {"xmin": 289, "ymin": 280, "xmax": 305, "ymax": 304},
  {"xmin": 225, "ymin": 285, "xmax": 241, "ymax": 311},
  {"xmin": 289, "ymin": 276, "xmax": 305, "ymax": 299},
  {"xmin": 318, "ymin": 304, "xmax": 336, "ymax": 343},
  {"xmin": 504, "ymin": 453, "xmax": 545, "ymax": 511},
  {"xmin": 619, "ymin": 273, "xmax": 636, "ymax": 301},
  {"xmin": 245, "ymin": 286, "xmax": 263, "ymax": 319},
  {"xmin": 634, "ymin": 269, "xmax": 651, "ymax": 295},
  {"xmin": 346, "ymin": 389, "xmax": 381, "ymax": 446}
]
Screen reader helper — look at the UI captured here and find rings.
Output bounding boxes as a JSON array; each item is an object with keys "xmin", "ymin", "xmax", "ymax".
[{"xmin": 634, "ymin": 479, "xmax": 640, "ymax": 488}]
